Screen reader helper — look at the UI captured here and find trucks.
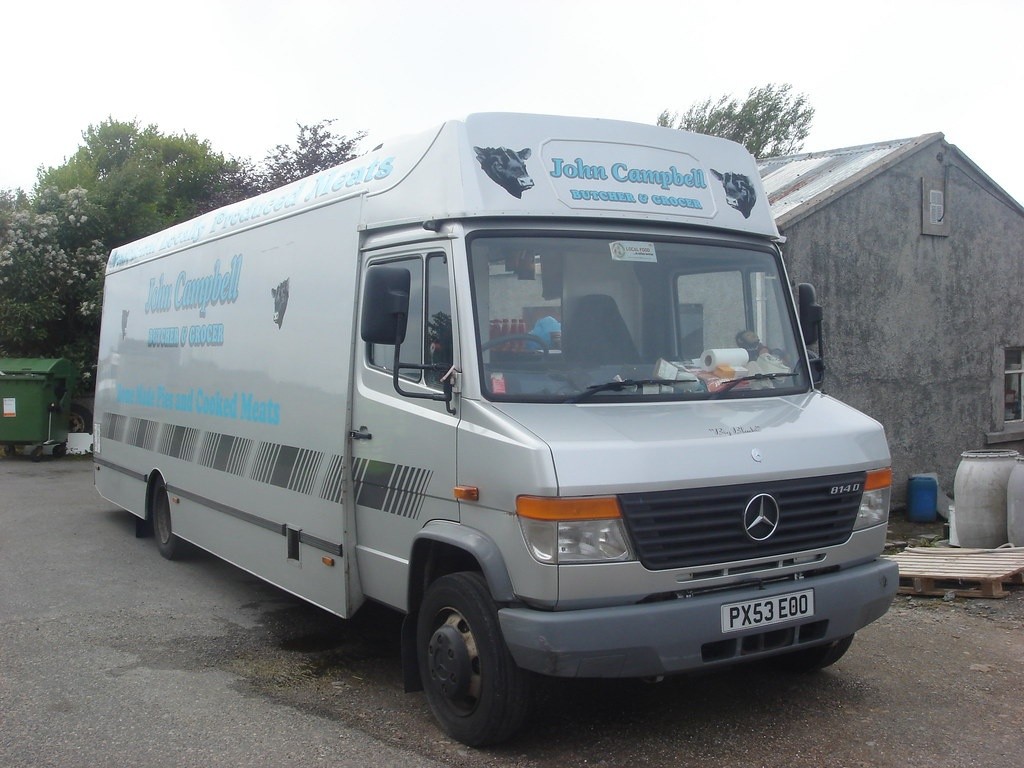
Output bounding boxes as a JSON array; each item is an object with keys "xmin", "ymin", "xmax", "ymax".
[{"xmin": 93, "ymin": 110, "xmax": 903, "ymax": 747}]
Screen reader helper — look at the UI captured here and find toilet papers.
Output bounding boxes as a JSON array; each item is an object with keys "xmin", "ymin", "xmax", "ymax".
[{"xmin": 700, "ymin": 349, "xmax": 749, "ymax": 372}]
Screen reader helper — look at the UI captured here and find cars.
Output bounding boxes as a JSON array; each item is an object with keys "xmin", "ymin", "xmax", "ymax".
[{"xmin": 71, "ymin": 364, "xmax": 97, "ymax": 434}]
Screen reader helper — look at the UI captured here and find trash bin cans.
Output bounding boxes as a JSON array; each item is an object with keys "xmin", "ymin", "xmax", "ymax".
[{"xmin": 0, "ymin": 358, "xmax": 77, "ymax": 462}]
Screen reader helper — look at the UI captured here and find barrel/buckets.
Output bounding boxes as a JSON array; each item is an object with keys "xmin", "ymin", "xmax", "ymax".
[
  {"xmin": 948, "ymin": 505, "xmax": 960, "ymax": 547},
  {"xmin": 953, "ymin": 449, "xmax": 1020, "ymax": 548},
  {"xmin": 905, "ymin": 476, "xmax": 938, "ymax": 522},
  {"xmin": 1007, "ymin": 455, "xmax": 1024, "ymax": 547}
]
